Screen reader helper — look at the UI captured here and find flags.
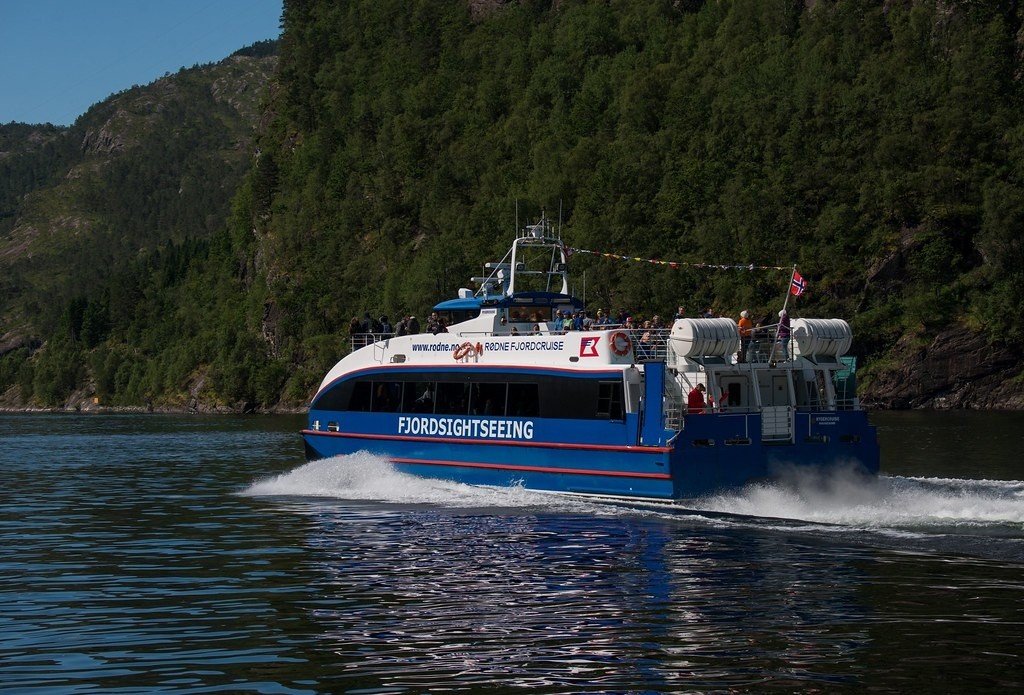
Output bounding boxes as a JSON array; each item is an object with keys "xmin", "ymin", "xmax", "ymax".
[{"xmin": 791, "ymin": 270, "xmax": 808, "ymax": 296}]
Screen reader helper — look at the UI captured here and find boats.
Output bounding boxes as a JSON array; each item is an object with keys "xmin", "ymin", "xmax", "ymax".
[{"xmin": 296, "ymin": 195, "xmax": 881, "ymax": 516}]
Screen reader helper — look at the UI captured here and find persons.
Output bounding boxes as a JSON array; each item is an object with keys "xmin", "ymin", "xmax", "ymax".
[
  {"xmin": 704, "ymin": 308, "xmax": 715, "ymax": 318},
  {"xmin": 509, "ymin": 306, "xmax": 689, "ymax": 359},
  {"xmin": 779, "ymin": 310, "xmax": 790, "ymax": 363},
  {"xmin": 738, "ymin": 310, "xmax": 774, "ymax": 363},
  {"xmin": 688, "ymin": 383, "xmax": 707, "ymax": 413},
  {"xmin": 349, "ymin": 312, "xmax": 449, "ymax": 350}
]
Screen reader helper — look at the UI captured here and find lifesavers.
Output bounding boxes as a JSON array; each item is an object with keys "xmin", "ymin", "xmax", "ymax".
[
  {"xmin": 454, "ymin": 341, "xmax": 472, "ymax": 360},
  {"xmin": 609, "ymin": 332, "xmax": 630, "ymax": 358}
]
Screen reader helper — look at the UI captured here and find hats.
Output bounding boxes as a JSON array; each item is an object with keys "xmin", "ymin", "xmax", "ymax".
[
  {"xmin": 698, "ymin": 384, "xmax": 705, "ymax": 391},
  {"xmin": 557, "ymin": 310, "xmax": 561, "ymax": 314},
  {"xmin": 741, "ymin": 311, "xmax": 749, "ymax": 318},
  {"xmin": 564, "ymin": 311, "xmax": 570, "ymax": 315},
  {"xmin": 626, "ymin": 317, "xmax": 633, "ymax": 322}
]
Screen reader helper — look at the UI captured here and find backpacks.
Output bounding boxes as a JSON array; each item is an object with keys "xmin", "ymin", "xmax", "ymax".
[
  {"xmin": 367, "ymin": 319, "xmax": 379, "ymax": 333},
  {"xmin": 383, "ymin": 323, "xmax": 390, "ymax": 336}
]
